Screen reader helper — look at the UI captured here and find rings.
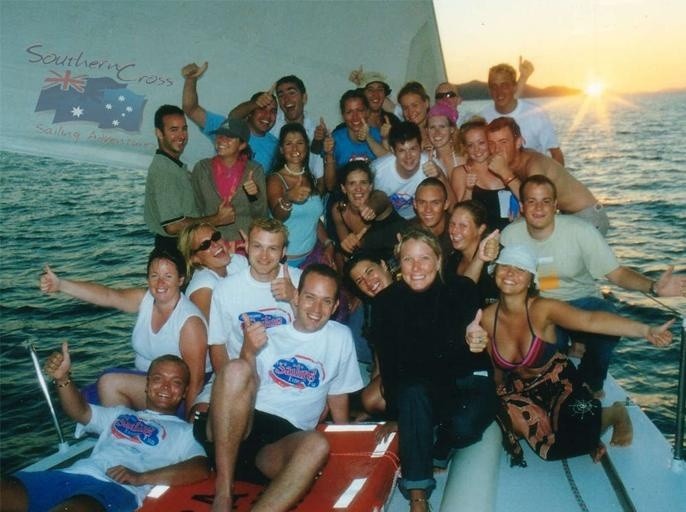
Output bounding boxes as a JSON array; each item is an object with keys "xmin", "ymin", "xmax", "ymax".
[
  {"xmin": 347, "ymin": 304, "xmax": 351, "ymax": 310},
  {"xmin": 479, "ymin": 338, "xmax": 482, "ymax": 343},
  {"xmin": 331, "ymin": 258, "xmax": 336, "ymax": 263}
]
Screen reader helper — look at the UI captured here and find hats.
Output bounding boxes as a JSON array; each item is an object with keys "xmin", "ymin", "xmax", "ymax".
[
  {"xmin": 208, "ymin": 120, "xmax": 251, "ymax": 138},
  {"xmin": 355, "ymin": 70, "xmax": 391, "ymax": 94},
  {"xmin": 486, "ymin": 246, "xmax": 539, "ymax": 279}
]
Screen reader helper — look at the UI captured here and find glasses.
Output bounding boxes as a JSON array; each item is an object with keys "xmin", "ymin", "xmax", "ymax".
[
  {"xmin": 190, "ymin": 231, "xmax": 224, "ymax": 257},
  {"xmin": 435, "ymin": 92, "xmax": 460, "ymax": 101}
]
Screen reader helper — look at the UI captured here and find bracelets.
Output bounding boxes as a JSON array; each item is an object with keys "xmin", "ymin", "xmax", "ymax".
[
  {"xmin": 324, "ymin": 155, "xmax": 337, "ymax": 164},
  {"xmin": 53, "ymin": 377, "xmax": 71, "ymax": 388},
  {"xmin": 503, "ymin": 175, "xmax": 519, "ymax": 186},
  {"xmin": 324, "ymin": 238, "xmax": 336, "ymax": 247},
  {"xmin": 279, "ymin": 198, "xmax": 293, "ymax": 211}
]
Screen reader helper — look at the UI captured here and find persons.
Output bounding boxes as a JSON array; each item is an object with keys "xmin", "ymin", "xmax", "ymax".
[
  {"xmin": 393, "ymin": 200, "xmax": 488, "ymax": 302},
  {"xmin": 423, "ymin": 99, "xmax": 468, "ymax": 166},
  {"xmin": 488, "ymin": 117, "xmax": 608, "ymax": 236},
  {"xmin": 380, "ymin": 80, "xmax": 435, "ymax": 152},
  {"xmin": 499, "ymin": 175, "xmax": 686, "ymax": 399},
  {"xmin": 194, "ymin": 263, "xmax": 362, "ymax": 512},
  {"xmin": 369, "ymin": 122, "xmax": 458, "ymax": 219},
  {"xmin": 209, "ymin": 219, "xmax": 330, "ymax": 423},
  {"xmin": 480, "ymin": 245, "xmax": 633, "ymax": 468},
  {"xmin": 348, "ymin": 55, "xmax": 534, "ymax": 129},
  {"xmin": 340, "ymin": 177, "xmax": 452, "ymax": 260},
  {"xmin": 41, "ymin": 247, "xmax": 210, "ymax": 421},
  {"xmin": 451, "ymin": 116, "xmax": 521, "ymax": 232},
  {"xmin": 371, "ymin": 231, "xmax": 489, "ymax": 512},
  {"xmin": 324, "ymin": 89, "xmax": 386, "ymax": 193},
  {"xmin": 192, "ymin": 119, "xmax": 268, "ymax": 256},
  {"xmin": 0, "ymin": 342, "xmax": 212, "ymax": 512},
  {"xmin": 326, "ymin": 160, "xmax": 408, "ymax": 315},
  {"xmin": 267, "ymin": 124, "xmax": 338, "ymax": 272},
  {"xmin": 311, "ymin": 73, "xmax": 402, "ymax": 153},
  {"xmin": 182, "ymin": 51, "xmax": 281, "ymax": 174},
  {"xmin": 179, "ymin": 223, "xmax": 249, "ymax": 323},
  {"xmin": 477, "ymin": 64, "xmax": 564, "ymax": 167},
  {"xmin": 145, "ymin": 106, "xmax": 236, "ymax": 247},
  {"xmin": 342, "ymin": 228, "xmax": 500, "ymax": 424},
  {"xmin": 227, "ymin": 76, "xmax": 326, "ymax": 195}
]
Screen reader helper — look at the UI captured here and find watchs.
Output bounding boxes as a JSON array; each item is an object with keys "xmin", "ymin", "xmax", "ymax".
[{"xmin": 650, "ymin": 281, "xmax": 658, "ymax": 298}]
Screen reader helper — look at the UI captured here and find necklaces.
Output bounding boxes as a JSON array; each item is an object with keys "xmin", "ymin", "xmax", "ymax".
[{"xmin": 284, "ymin": 163, "xmax": 305, "ymax": 176}]
